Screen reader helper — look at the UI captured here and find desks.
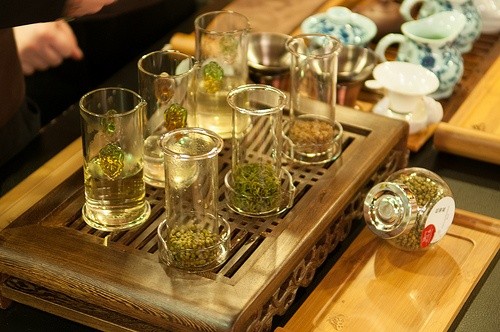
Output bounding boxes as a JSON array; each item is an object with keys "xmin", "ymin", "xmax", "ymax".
[{"xmin": 0, "ymin": 0, "xmax": 500, "ymax": 332}]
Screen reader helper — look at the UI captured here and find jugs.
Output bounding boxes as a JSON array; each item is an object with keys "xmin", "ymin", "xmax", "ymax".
[
  {"xmin": 399, "ymin": 0, "xmax": 482, "ymax": 54},
  {"xmin": 374, "ymin": 11, "xmax": 466, "ymax": 99}
]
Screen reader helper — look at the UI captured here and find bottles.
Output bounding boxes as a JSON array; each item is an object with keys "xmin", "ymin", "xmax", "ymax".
[{"xmin": 363, "ymin": 167, "xmax": 455, "ymax": 251}]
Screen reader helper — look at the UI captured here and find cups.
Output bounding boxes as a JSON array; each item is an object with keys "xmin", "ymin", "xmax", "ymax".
[
  {"xmin": 195, "ymin": 11, "xmax": 250, "ymax": 140},
  {"xmin": 372, "ymin": 61, "xmax": 440, "ymax": 113},
  {"xmin": 301, "ymin": 6, "xmax": 377, "ymax": 75},
  {"xmin": 283, "ymin": 34, "xmax": 343, "ymax": 165},
  {"xmin": 244, "ymin": 32, "xmax": 293, "ymax": 91},
  {"xmin": 139, "ymin": 49, "xmax": 199, "ymax": 188},
  {"xmin": 158, "ymin": 127, "xmax": 230, "ymax": 272},
  {"xmin": 337, "ymin": 44, "xmax": 377, "ymax": 108},
  {"xmin": 224, "ymin": 84, "xmax": 296, "ymax": 218},
  {"xmin": 78, "ymin": 88, "xmax": 151, "ymax": 231}
]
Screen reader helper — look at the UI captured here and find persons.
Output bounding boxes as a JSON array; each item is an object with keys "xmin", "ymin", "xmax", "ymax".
[{"xmin": 0, "ymin": 0, "xmax": 117, "ymax": 173}]
